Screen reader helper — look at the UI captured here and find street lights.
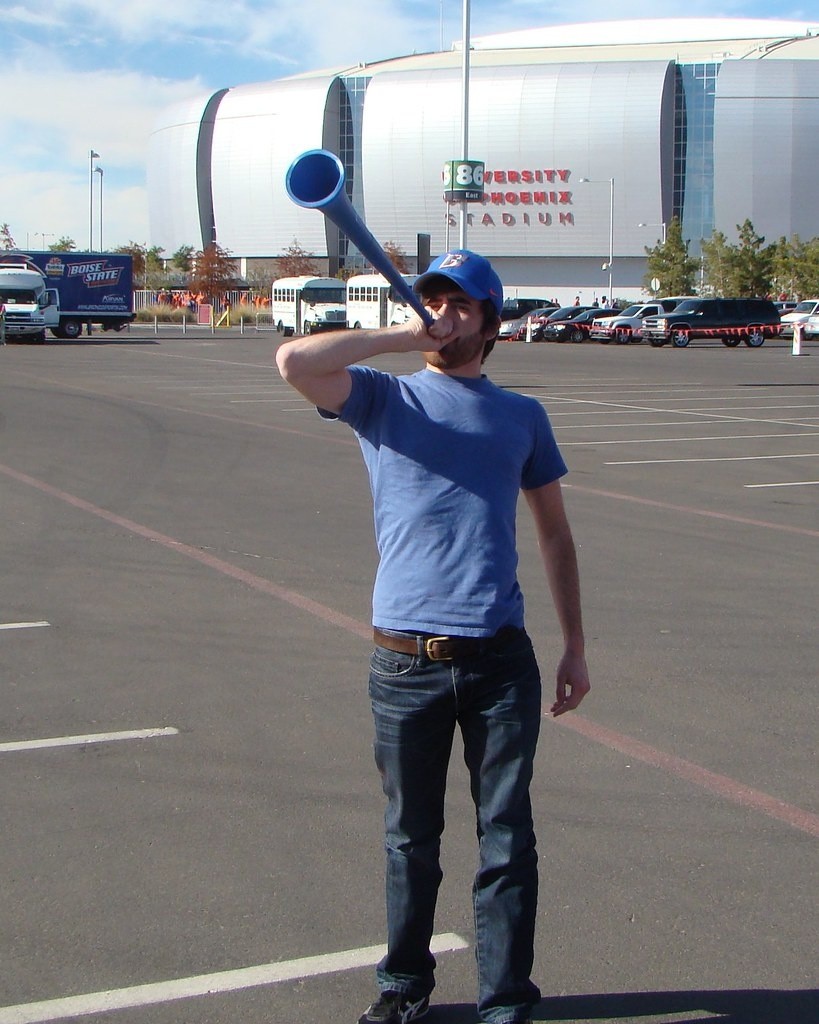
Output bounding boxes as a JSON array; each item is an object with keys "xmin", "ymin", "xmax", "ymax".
[
  {"xmin": 577, "ymin": 176, "xmax": 614, "ymax": 308},
  {"xmin": 87, "ymin": 147, "xmax": 102, "ymax": 253},
  {"xmin": 638, "ymin": 222, "xmax": 666, "ymax": 263},
  {"xmin": 92, "ymin": 165, "xmax": 104, "ymax": 255}
]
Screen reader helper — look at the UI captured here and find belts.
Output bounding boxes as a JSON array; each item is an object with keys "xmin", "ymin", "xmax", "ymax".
[{"xmin": 374, "ymin": 626, "xmax": 515, "ymax": 660}]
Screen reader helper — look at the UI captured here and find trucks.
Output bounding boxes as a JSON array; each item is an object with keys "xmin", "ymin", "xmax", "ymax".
[
  {"xmin": 0, "ymin": 248, "xmax": 138, "ymax": 341},
  {"xmin": 346, "ymin": 271, "xmax": 423, "ymax": 331},
  {"xmin": 0, "ymin": 268, "xmax": 61, "ymax": 344},
  {"xmin": 272, "ymin": 274, "xmax": 347, "ymax": 337}
]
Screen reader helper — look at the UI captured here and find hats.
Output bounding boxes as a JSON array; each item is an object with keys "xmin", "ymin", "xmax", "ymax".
[{"xmin": 412, "ymin": 250, "xmax": 503, "ymax": 316}]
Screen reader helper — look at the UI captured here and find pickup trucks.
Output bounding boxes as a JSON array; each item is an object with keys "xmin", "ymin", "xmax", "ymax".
[
  {"xmin": 500, "ymin": 298, "xmax": 558, "ymax": 323},
  {"xmin": 588, "ymin": 294, "xmax": 700, "ymax": 346}
]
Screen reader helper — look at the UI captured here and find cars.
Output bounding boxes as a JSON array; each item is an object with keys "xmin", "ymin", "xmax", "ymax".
[
  {"xmin": 771, "ymin": 300, "xmax": 819, "ymax": 341},
  {"xmin": 518, "ymin": 305, "xmax": 603, "ymax": 343},
  {"xmin": 497, "ymin": 305, "xmax": 558, "ymax": 342},
  {"xmin": 542, "ymin": 307, "xmax": 623, "ymax": 344}
]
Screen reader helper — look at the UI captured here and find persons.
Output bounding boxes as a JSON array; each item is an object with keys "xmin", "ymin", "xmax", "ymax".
[{"xmin": 274, "ymin": 253, "xmax": 591, "ymax": 1024}]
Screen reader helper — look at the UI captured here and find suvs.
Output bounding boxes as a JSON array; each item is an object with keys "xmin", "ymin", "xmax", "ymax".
[{"xmin": 640, "ymin": 296, "xmax": 782, "ymax": 348}]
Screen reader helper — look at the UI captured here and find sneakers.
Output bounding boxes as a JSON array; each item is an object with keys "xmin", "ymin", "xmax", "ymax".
[{"xmin": 356, "ymin": 994, "xmax": 429, "ymax": 1024}]
